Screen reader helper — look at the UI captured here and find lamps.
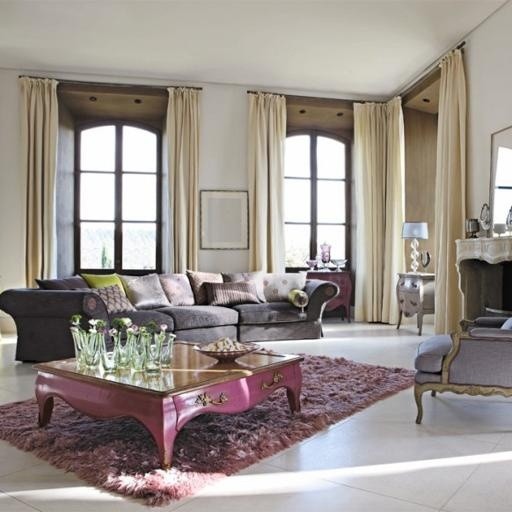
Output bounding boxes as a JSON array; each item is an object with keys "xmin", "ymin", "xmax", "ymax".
[{"xmin": 400, "ymin": 220, "xmax": 429, "ymax": 274}]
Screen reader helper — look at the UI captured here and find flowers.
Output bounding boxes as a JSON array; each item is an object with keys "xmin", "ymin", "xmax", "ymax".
[{"xmin": 67, "ymin": 309, "xmax": 175, "ymax": 374}]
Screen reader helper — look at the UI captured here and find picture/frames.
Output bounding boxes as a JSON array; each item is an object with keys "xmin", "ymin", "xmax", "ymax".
[{"xmin": 199, "ymin": 188, "xmax": 250, "ymax": 250}]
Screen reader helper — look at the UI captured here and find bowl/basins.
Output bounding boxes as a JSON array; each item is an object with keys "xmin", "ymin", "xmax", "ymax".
[{"xmin": 191, "ymin": 341, "xmax": 261, "ymax": 363}]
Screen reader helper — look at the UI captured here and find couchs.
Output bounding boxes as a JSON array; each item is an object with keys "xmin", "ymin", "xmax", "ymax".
[{"xmin": 0, "ymin": 268, "xmax": 342, "ymax": 363}]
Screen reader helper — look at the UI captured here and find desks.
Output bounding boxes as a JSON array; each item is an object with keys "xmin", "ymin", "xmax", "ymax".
[
  {"xmin": 302, "ymin": 269, "xmax": 352, "ymax": 325},
  {"xmin": 395, "ymin": 272, "xmax": 435, "ymax": 336}
]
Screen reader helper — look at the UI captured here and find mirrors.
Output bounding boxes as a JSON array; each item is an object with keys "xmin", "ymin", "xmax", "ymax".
[{"xmin": 485, "ymin": 128, "xmax": 511, "ymax": 237}]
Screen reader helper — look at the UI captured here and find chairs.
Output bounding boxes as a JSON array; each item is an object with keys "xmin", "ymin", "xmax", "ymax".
[{"xmin": 411, "ymin": 307, "xmax": 512, "ymax": 425}]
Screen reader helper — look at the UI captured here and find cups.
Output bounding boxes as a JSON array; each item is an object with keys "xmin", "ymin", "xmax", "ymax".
[{"xmin": 316, "ymin": 256, "xmax": 325, "ymax": 269}]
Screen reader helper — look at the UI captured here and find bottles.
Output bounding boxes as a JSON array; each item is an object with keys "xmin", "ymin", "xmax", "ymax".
[
  {"xmin": 467, "ymin": 218, "xmax": 479, "ymax": 233},
  {"xmin": 321, "ymin": 244, "xmax": 331, "ymax": 263}
]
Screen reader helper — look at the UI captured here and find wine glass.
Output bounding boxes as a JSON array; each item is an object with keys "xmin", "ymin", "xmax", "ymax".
[
  {"xmin": 305, "ymin": 259, "xmax": 319, "ymax": 272},
  {"xmin": 330, "ymin": 258, "xmax": 348, "ymax": 271}
]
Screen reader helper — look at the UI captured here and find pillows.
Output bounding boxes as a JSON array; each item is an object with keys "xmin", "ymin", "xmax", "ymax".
[{"xmin": 34, "ymin": 267, "xmax": 308, "ymax": 316}]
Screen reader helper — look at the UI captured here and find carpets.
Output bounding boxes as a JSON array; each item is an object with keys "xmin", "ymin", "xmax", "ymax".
[{"xmin": 0, "ymin": 352, "xmax": 422, "ymax": 509}]
